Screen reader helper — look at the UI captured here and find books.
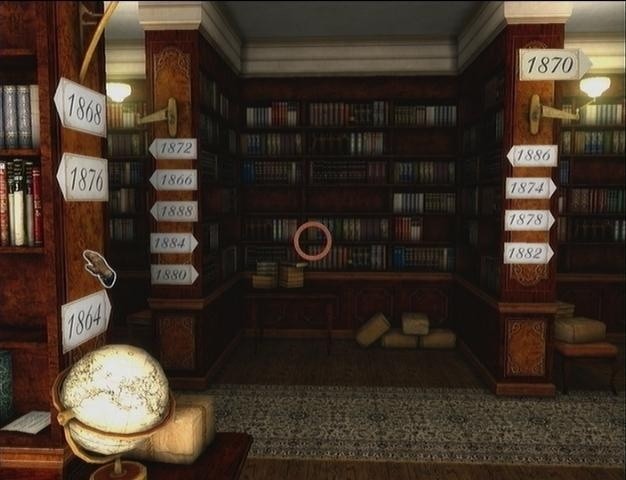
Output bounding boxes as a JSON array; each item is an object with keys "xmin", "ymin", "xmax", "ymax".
[
  {"xmin": 101, "ymin": 101, "xmax": 151, "ymax": 242},
  {"xmin": 555, "ymin": 97, "xmax": 626, "ymax": 245},
  {"xmin": 0, "ymin": 78, "xmax": 47, "ymax": 251}
]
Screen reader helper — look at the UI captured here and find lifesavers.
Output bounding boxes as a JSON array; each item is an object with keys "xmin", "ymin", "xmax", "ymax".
[{"xmin": 294, "ymin": 222, "xmax": 332, "ymax": 261}]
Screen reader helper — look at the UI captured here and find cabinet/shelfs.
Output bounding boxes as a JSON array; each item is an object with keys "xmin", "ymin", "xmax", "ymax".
[
  {"xmin": 559, "ymin": 73, "xmax": 626, "ymax": 278},
  {"xmin": 190, "ymin": 28, "xmax": 236, "ymax": 307},
  {"xmin": 103, "ymin": 75, "xmax": 153, "ymax": 271},
  {"xmin": 463, "ymin": 28, "xmax": 515, "ymax": 306},
  {"xmin": 236, "ymin": 74, "xmax": 460, "ymax": 274},
  {"xmin": 0, "ymin": 0, "xmax": 106, "ymax": 451}
]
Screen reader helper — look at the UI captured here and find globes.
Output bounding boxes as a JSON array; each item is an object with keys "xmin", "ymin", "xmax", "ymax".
[{"xmin": 51, "ymin": 345, "xmax": 176, "ymax": 480}]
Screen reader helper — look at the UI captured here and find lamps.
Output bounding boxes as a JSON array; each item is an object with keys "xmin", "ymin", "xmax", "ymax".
[
  {"xmin": 581, "ymin": 71, "xmax": 612, "ymax": 101},
  {"xmin": 107, "ymin": 82, "xmax": 135, "ymax": 108}
]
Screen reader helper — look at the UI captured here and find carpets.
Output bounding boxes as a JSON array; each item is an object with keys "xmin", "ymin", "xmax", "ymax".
[{"xmin": 184, "ymin": 380, "xmax": 625, "ymax": 466}]
